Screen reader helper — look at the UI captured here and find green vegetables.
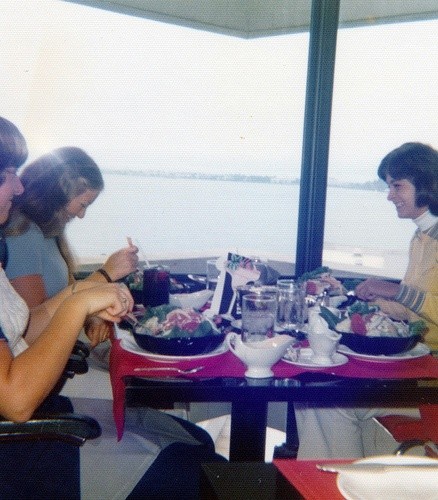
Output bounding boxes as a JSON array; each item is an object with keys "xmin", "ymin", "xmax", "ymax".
[
  {"xmin": 407, "ymin": 320, "xmax": 424, "ymax": 335},
  {"xmin": 133, "ymin": 305, "xmax": 220, "ymax": 338},
  {"xmin": 124, "ymin": 272, "xmax": 144, "ymax": 290},
  {"xmin": 297, "ymin": 265, "xmax": 329, "ymax": 285},
  {"xmin": 319, "ymin": 301, "xmax": 379, "ymax": 328}
]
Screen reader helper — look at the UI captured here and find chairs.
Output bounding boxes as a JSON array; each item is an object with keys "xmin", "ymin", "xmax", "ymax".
[{"xmin": 0, "ymin": 355, "xmax": 102, "ymax": 500}]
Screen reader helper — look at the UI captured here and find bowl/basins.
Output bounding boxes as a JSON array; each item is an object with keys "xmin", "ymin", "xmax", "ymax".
[
  {"xmin": 335, "ymin": 331, "xmax": 420, "ymax": 356},
  {"xmin": 315, "ymin": 294, "xmax": 360, "ymax": 309},
  {"xmin": 169, "ymin": 288, "xmax": 211, "ymax": 312},
  {"xmin": 132, "ymin": 323, "xmax": 224, "ymax": 355},
  {"xmin": 129, "ymin": 273, "xmax": 207, "ymax": 303}
]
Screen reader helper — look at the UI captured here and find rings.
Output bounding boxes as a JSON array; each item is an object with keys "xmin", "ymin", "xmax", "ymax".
[{"xmin": 121, "ymin": 297, "xmax": 127, "ymax": 304}]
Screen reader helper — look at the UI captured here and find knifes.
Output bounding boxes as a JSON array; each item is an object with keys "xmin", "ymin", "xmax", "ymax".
[{"xmin": 316, "ymin": 463, "xmax": 438, "ymax": 473}]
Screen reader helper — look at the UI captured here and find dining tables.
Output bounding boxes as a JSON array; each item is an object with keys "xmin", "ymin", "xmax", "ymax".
[{"xmin": 110, "ymin": 291, "xmax": 438, "ymax": 463}]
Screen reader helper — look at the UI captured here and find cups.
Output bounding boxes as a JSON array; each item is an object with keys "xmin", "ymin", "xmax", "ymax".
[
  {"xmin": 243, "ymin": 258, "xmax": 305, "ymax": 341},
  {"xmin": 142, "ymin": 269, "xmax": 170, "ymax": 308},
  {"xmin": 205, "ymin": 260, "xmax": 221, "ymax": 302}
]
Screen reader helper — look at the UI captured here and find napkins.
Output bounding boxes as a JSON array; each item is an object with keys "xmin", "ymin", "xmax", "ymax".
[{"xmin": 70, "ymin": 397, "xmax": 203, "ymax": 500}]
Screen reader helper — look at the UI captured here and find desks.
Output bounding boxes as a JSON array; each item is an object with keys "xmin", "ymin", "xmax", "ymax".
[{"xmin": 203, "ymin": 460, "xmax": 358, "ymax": 500}]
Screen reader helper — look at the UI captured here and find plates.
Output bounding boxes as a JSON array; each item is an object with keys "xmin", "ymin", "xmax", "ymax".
[
  {"xmin": 230, "ymin": 318, "xmax": 286, "ymax": 333},
  {"xmin": 336, "ymin": 455, "xmax": 438, "ymax": 500},
  {"xmin": 118, "ymin": 334, "xmax": 228, "ymax": 363},
  {"xmin": 336, "ymin": 342, "xmax": 430, "ymax": 362},
  {"xmin": 281, "ymin": 347, "xmax": 349, "ymax": 367}
]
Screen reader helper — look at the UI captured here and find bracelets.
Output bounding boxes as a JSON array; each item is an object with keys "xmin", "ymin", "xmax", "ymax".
[
  {"xmin": 97, "ymin": 269, "xmax": 113, "ymax": 283},
  {"xmin": 71, "ymin": 279, "xmax": 78, "ymax": 293}
]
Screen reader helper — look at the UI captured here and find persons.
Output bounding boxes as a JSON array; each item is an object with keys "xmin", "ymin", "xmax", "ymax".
[
  {"xmin": 0, "ymin": 117, "xmax": 215, "ymax": 500},
  {"xmin": 293, "ymin": 142, "xmax": 438, "ymax": 460},
  {"xmin": 0, "ymin": 146, "xmax": 139, "ymax": 370}
]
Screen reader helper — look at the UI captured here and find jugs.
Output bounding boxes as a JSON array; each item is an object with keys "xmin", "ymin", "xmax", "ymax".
[
  {"xmin": 226, "ymin": 331, "xmax": 296, "ymax": 379},
  {"xmin": 308, "ymin": 313, "xmax": 343, "ymax": 364}
]
